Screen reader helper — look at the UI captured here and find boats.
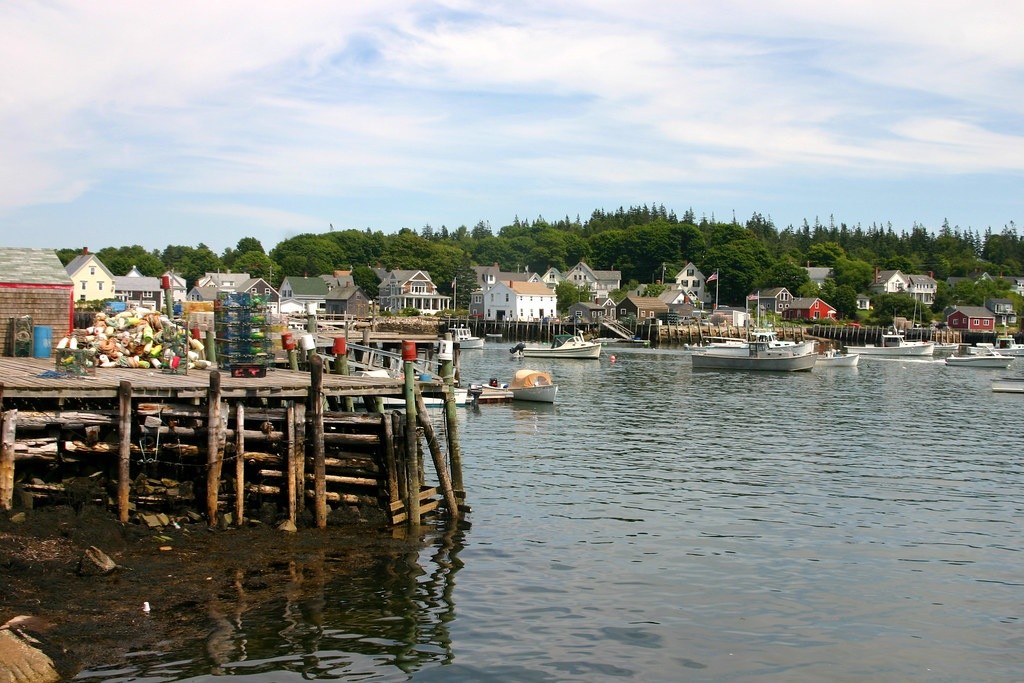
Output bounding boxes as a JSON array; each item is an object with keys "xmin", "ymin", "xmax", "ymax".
[
  {"xmin": 813, "ymin": 349, "xmax": 861, "ymax": 368},
  {"xmin": 448, "ymin": 325, "xmax": 485, "ymax": 349},
  {"xmin": 337, "ymin": 370, "xmax": 469, "ymax": 407},
  {"xmin": 935, "ymin": 341, "xmax": 960, "ymax": 351},
  {"xmin": 500, "ymin": 368, "xmax": 560, "ymax": 404},
  {"xmin": 691, "ymin": 328, "xmax": 819, "ymax": 371},
  {"xmin": 519, "ymin": 334, "xmax": 602, "ymax": 358},
  {"xmin": 843, "ymin": 324, "xmax": 936, "ymax": 357},
  {"xmin": 992, "ymin": 336, "xmax": 1024, "ymax": 356},
  {"xmin": 989, "ymin": 375, "xmax": 1024, "ymax": 394},
  {"xmin": 945, "ymin": 344, "xmax": 1016, "ymax": 369}
]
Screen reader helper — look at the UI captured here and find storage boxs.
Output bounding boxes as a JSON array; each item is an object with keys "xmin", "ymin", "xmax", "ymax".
[{"xmin": 229, "ymin": 365, "xmax": 267, "ymax": 378}]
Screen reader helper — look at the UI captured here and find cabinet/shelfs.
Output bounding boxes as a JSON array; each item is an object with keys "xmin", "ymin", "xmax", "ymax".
[{"xmin": 215, "ymin": 306, "xmax": 275, "ymax": 371}]
